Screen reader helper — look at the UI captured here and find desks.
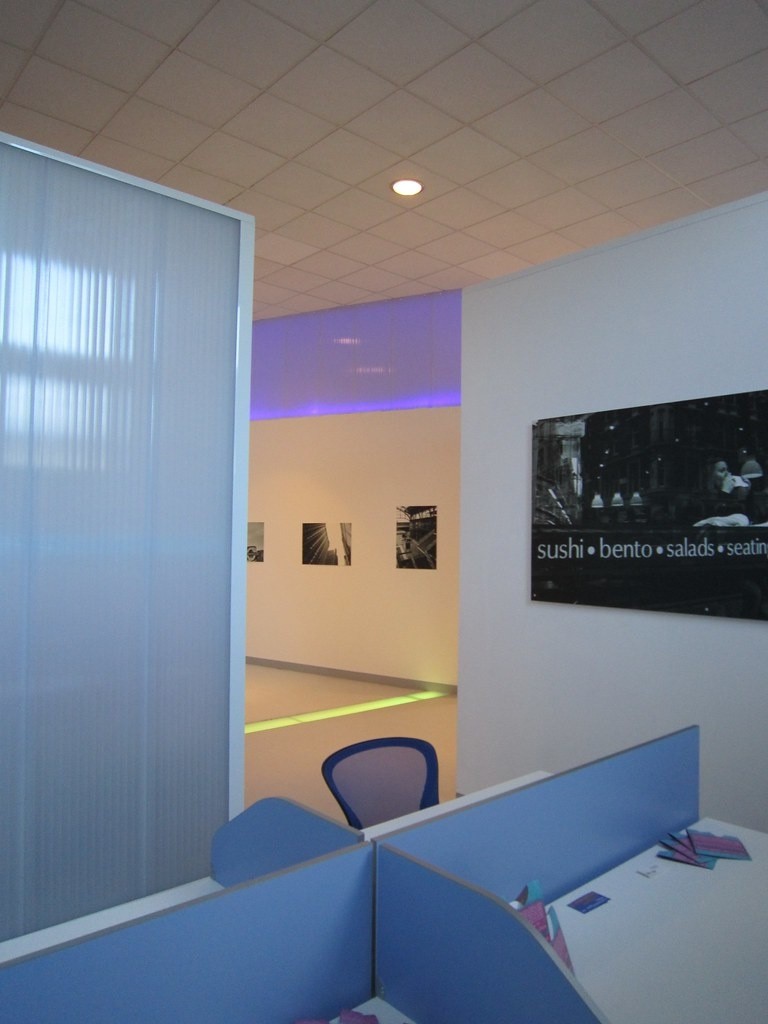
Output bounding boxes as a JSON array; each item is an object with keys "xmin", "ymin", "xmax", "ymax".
[{"xmin": 0, "ymin": 771, "xmax": 768, "ymax": 1024}]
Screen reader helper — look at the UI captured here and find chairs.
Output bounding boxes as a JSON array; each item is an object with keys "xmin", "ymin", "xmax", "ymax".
[{"xmin": 321, "ymin": 737, "xmax": 440, "ymax": 830}]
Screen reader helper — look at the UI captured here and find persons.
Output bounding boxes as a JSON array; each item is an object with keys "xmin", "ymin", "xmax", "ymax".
[{"xmin": 700, "ymin": 458, "xmax": 752, "ymax": 517}]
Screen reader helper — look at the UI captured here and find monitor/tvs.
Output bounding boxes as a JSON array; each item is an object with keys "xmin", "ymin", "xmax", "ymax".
[{"xmin": 530, "ymin": 390, "xmax": 768, "ymax": 621}]
